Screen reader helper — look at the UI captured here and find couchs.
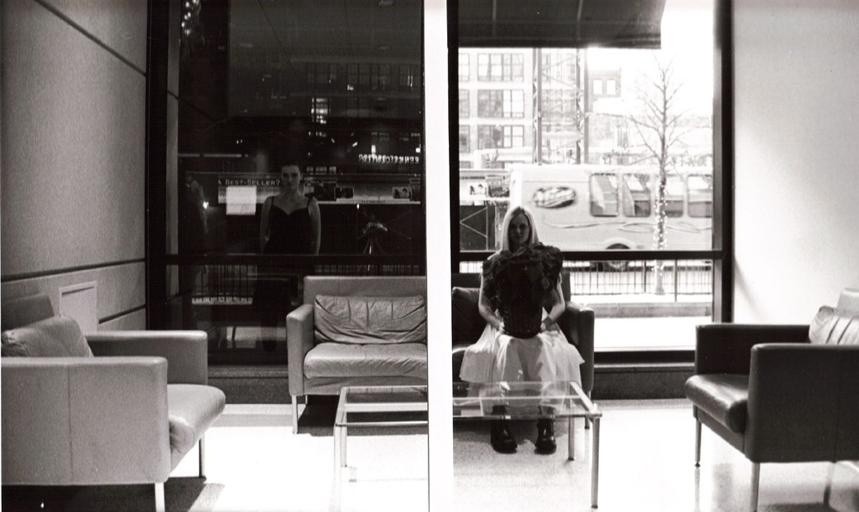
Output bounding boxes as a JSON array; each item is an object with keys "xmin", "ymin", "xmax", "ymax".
[{"xmin": 285, "ymin": 274, "xmax": 596, "ymax": 436}]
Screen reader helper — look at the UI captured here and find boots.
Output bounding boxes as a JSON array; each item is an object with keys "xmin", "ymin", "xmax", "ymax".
[
  {"xmin": 533, "ymin": 405, "xmax": 558, "ymax": 456},
  {"xmin": 490, "ymin": 404, "xmax": 520, "ymax": 455}
]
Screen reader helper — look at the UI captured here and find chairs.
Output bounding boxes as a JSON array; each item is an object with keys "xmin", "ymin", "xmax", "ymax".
[
  {"xmin": 682, "ymin": 285, "xmax": 858, "ymax": 511},
  {"xmin": 2, "ymin": 289, "xmax": 228, "ymax": 512}
]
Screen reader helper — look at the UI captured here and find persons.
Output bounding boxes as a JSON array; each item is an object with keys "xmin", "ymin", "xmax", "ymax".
[
  {"xmin": 181, "ymin": 156, "xmax": 208, "ymax": 303},
  {"xmin": 256, "ymin": 161, "xmax": 322, "ymax": 312},
  {"xmin": 459, "ymin": 204, "xmax": 585, "ymax": 452}
]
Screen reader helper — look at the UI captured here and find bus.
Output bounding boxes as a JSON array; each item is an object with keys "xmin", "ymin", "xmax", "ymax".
[
  {"xmin": 500, "ymin": 157, "xmax": 712, "ymax": 273},
  {"xmin": 457, "ymin": 167, "xmax": 506, "ymax": 253}
]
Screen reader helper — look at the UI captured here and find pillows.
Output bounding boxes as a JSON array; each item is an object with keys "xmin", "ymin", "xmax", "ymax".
[
  {"xmin": 312, "ymin": 291, "xmax": 427, "ymax": 346},
  {"xmin": 452, "ymin": 285, "xmax": 488, "ymax": 346},
  {"xmin": 3, "ymin": 311, "xmax": 97, "ymax": 357},
  {"xmin": 807, "ymin": 304, "xmax": 859, "ymax": 345}
]
